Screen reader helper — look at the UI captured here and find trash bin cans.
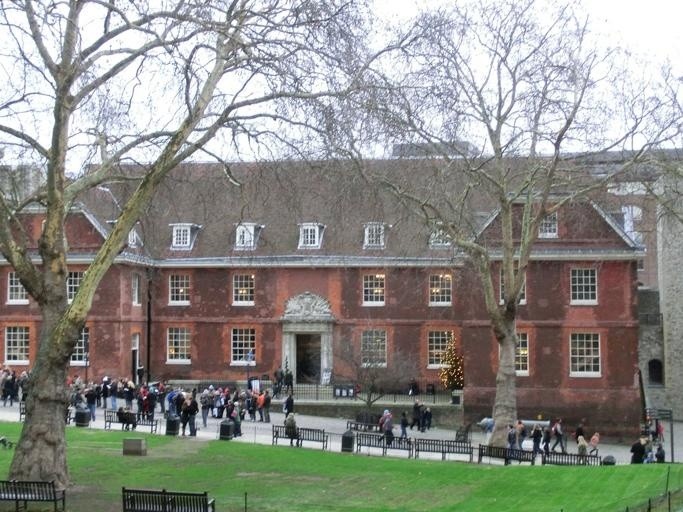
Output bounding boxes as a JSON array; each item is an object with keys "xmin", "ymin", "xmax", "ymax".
[
  {"xmin": 426, "ymin": 383, "xmax": 434, "ymax": 395},
  {"xmin": 342, "ymin": 431, "xmax": 355, "ymax": 452},
  {"xmin": 333, "ymin": 383, "xmax": 354, "ymax": 398},
  {"xmin": 166, "ymin": 416, "xmax": 180, "ymax": 435},
  {"xmin": 76, "ymin": 407, "xmax": 90, "ymax": 427},
  {"xmin": 248, "ymin": 376, "xmax": 257, "ymax": 389},
  {"xmin": 220, "ymin": 419, "xmax": 235, "ymax": 441}
]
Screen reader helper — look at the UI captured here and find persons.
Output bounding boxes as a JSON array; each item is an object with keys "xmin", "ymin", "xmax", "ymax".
[
  {"xmin": 66, "ymin": 375, "xmax": 199, "ymax": 437},
  {"xmin": 200, "ymin": 385, "xmax": 271, "ymax": 437},
  {"xmin": 284, "ymin": 413, "xmax": 300, "ymax": 448},
  {"xmin": 283, "ymin": 394, "xmax": 293, "ymax": 426},
  {"xmin": 407, "ymin": 376, "xmax": 419, "ymax": 395},
  {"xmin": 1, "ymin": 366, "xmax": 33, "ymax": 404},
  {"xmin": 629, "ymin": 414, "xmax": 666, "ymax": 464},
  {"xmin": 271, "ymin": 366, "xmax": 295, "ymax": 399},
  {"xmin": 473, "ymin": 413, "xmax": 601, "ymax": 465},
  {"xmin": 376, "ymin": 398, "xmax": 434, "ymax": 446}
]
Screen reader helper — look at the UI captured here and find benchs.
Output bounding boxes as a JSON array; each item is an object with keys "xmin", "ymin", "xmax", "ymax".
[
  {"xmin": 19, "ymin": 402, "xmax": 71, "ymax": 424},
  {"xmin": 104, "ymin": 411, "xmax": 158, "ymax": 434},
  {"xmin": 272, "ymin": 424, "xmax": 328, "ymax": 450},
  {"xmin": 121, "ymin": 486, "xmax": 215, "ymax": 512},
  {"xmin": 354, "ymin": 433, "xmax": 602, "ymax": 466},
  {"xmin": 0, "ymin": 479, "xmax": 66, "ymax": 512}
]
